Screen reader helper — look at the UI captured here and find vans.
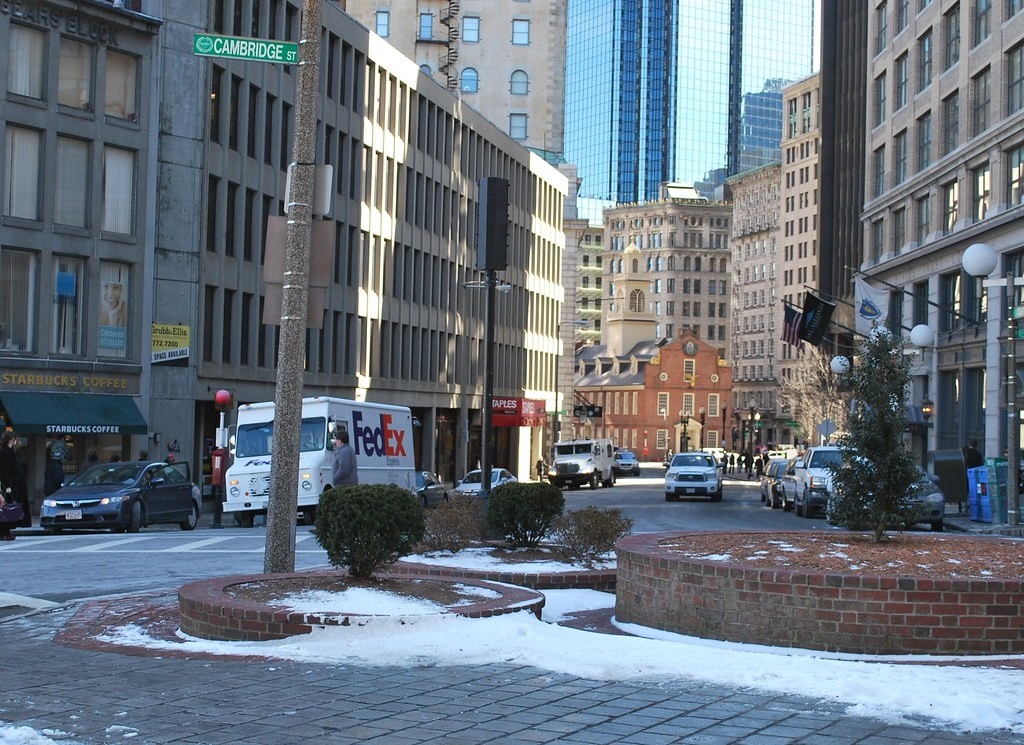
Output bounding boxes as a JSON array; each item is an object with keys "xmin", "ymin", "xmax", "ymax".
[
  {"xmin": 222, "ymin": 395, "xmax": 422, "ymax": 527},
  {"xmin": 548, "ymin": 437, "xmax": 614, "ymax": 490}
]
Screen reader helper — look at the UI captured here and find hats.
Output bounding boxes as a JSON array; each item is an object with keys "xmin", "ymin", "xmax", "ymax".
[{"xmin": 169, "ymin": 453, "xmax": 174, "ymax": 458}]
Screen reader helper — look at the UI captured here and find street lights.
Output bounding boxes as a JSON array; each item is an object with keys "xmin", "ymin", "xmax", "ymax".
[
  {"xmin": 829, "ymin": 323, "xmax": 933, "ymax": 414},
  {"xmin": 699, "ymin": 406, "xmax": 706, "ymax": 452},
  {"xmin": 679, "ymin": 409, "xmax": 690, "ymax": 452},
  {"xmin": 745, "ymin": 397, "xmax": 760, "ymax": 479},
  {"xmin": 963, "ymin": 242, "xmax": 1024, "ymax": 525},
  {"xmin": 721, "ymin": 399, "xmax": 727, "ymax": 448},
  {"xmin": 210, "ymin": 389, "xmax": 231, "ymax": 529}
]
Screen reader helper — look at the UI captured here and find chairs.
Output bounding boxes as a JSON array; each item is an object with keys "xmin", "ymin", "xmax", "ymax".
[{"xmin": 678, "ymin": 459, "xmax": 690, "ymax": 466}]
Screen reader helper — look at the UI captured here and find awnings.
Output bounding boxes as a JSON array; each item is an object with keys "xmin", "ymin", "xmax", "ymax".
[{"xmin": 0, "ymin": 390, "xmax": 149, "ymax": 437}]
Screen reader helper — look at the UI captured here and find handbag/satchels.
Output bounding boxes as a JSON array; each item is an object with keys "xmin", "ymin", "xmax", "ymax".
[{"xmin": 0, "ymin": 491, "xmax": 24, "ymax": 522}]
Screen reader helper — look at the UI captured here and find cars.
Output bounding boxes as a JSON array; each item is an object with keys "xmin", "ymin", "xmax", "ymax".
[
  {"xmin": 415, "ymin": 472, "xmax": 449, "ymax": 510},
  {"xmin": 759, "ymin": 446, "xmax": 946, "ymax": 531},
  {"xmin": 612, "ymin": 451, "xmax": 641, "ymax": 477},
  {"xmin": 455, "ymin": 467, "xmax": 517, "ymax": 497},
  {"xmin": 40, "ymin": 460, "xmax": 203, "ymax": 535},
  {"xmin": 663, "ymin": 452, "xmax": 724, "ymax": 502}
]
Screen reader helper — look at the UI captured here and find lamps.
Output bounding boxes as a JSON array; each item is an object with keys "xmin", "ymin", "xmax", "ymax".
[{"xmin": 112, "ymin": 0, "xmax": 123, "ymax": 8}]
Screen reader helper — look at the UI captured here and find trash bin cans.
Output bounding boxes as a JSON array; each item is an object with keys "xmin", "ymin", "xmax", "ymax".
[{"xmin": 967, "ymin": 465, "xmax": 1009, "ymax": 523}]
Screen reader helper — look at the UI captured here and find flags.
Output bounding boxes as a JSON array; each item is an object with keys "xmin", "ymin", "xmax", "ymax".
[
  {"xmin": 854, "ymin": 277, "xmax": 890, "ymax": 336},
  {"xmin": 780, "ymin": 303, "xmax": 803, "ymax": 349},
  {"xmin": 796, "ymin": 290, "xmax": 837, "ymax": 346}
]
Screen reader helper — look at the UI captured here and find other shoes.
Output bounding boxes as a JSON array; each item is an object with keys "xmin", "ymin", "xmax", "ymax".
[{"xmin": 0, "ymin": 535, "xmax": 15, "ymax": 541}]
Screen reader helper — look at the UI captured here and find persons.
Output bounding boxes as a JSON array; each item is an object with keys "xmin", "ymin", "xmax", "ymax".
[
  {"xmin": 0, "ymin": 430, "xmax": 21, "ymax": 541},
  {"xmin": 666, "ymin": 448, "xmax": 673, "ymax": 473},
  {"xmin": 536, "ymin": 456, "xmax": 552, "ymax": 483},
  {"xmin": 962, "ymin": 440, "xmax": 983, "ymax": 493},
  {"xmin": 44, "ymin": 451, "xmax": 65, "ymax": 497},
  {"xmin": 79, "ymin": 450, "xmax": 175, "ymax": 488},
  {"xmin": 722, "ymin": 445, "xmax": 771, "ymax": 482},
  {"xmin": 308, "ymin": 430, "xmax": 359, "ymax": 535}
]
